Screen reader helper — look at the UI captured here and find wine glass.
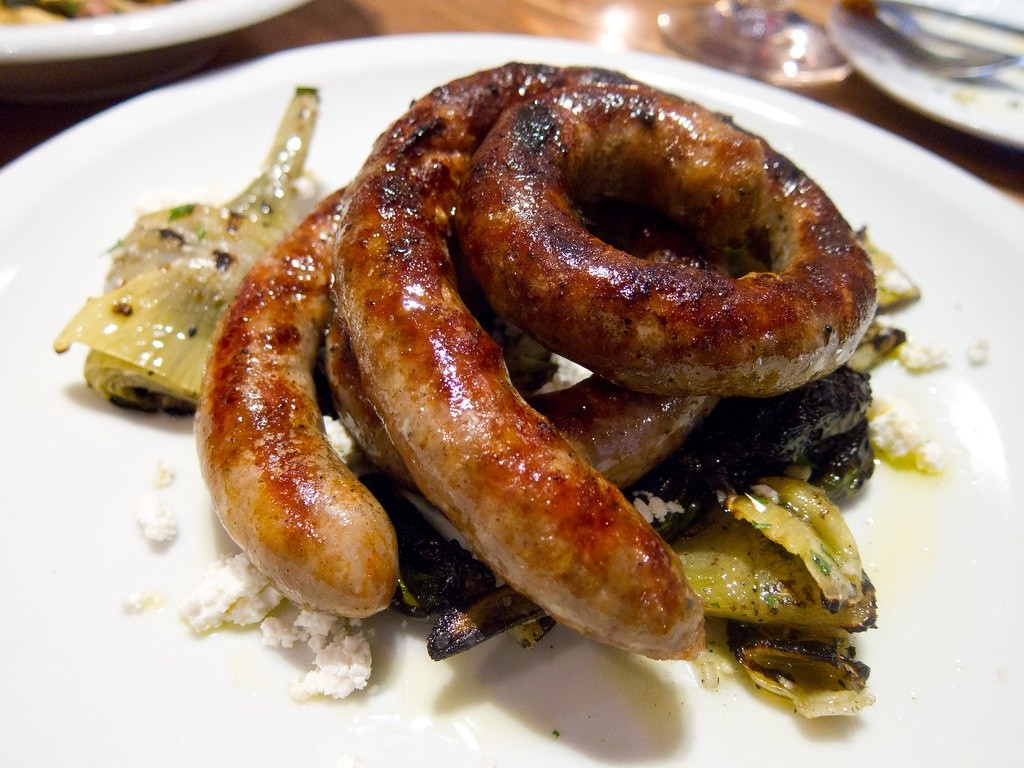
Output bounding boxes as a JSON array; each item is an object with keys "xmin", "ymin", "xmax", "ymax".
[{"xmin": 656, "ymin": 0, "xmax": 857, "ymax": 88}]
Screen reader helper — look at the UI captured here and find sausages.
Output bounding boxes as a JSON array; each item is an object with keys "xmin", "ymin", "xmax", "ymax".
[
  {"xmin": 193, "ymin": 185, "xmax": 719, "ymax": 614},
  {"xmin": 331, "ymin": 62, "xmax": 881, "ymax": 660}
]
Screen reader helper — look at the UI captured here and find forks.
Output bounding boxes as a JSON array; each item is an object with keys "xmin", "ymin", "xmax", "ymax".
[{"xmin": 841, "ymin": 0, "xmax": 1024, "ymax": 80}]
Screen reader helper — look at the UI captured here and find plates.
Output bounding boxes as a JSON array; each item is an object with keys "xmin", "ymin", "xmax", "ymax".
[
  {"xmin": 0, "ymin": 34, "xmax": 1024, "ymax": 768},
  {"xmin": 0, "ymin": 0, "xmax": 315, "ymax": 63},
  {"xmin": 823, "ymin": 0, "xmax": 1024, "ymax": 153}
]
